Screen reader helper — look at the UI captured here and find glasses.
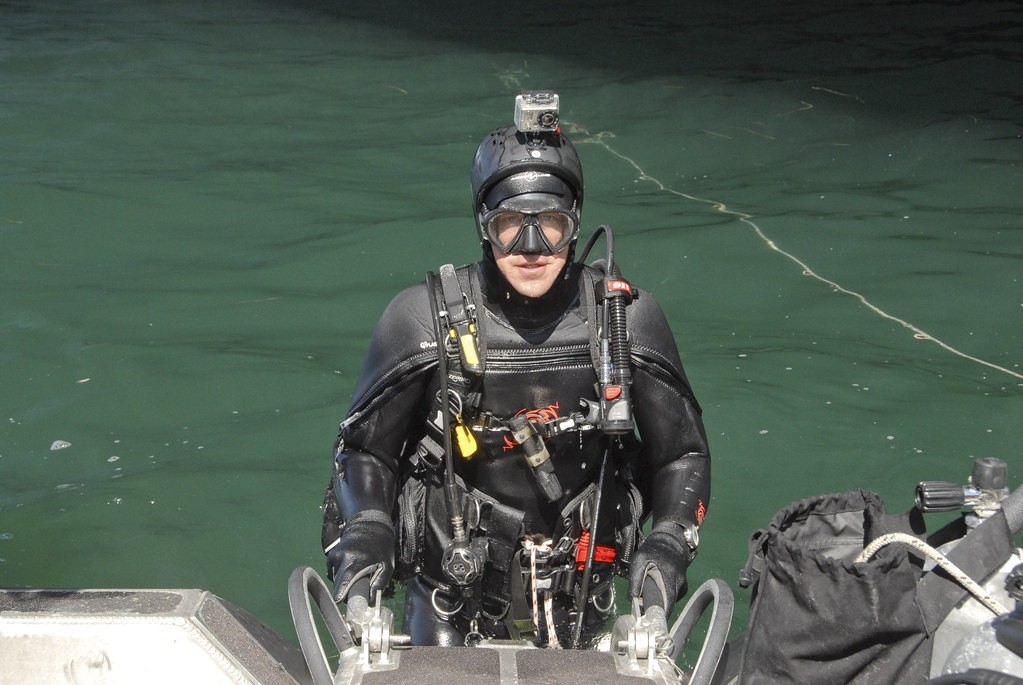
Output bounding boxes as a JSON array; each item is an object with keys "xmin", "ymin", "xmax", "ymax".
[{"xmin": 481, "ymin": 200, "xmax": 578, "ymax": 256}]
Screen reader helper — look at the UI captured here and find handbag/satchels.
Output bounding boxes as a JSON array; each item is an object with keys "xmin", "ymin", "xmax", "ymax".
[{"xmin": 736, "ymin": 490, "xmax": 1013, "ymax": 685}]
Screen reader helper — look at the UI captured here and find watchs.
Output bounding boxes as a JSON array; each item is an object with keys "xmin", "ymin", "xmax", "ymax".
[{"xmin": 654, "ymin": 514, "xmax": 699, "ymax": 550}]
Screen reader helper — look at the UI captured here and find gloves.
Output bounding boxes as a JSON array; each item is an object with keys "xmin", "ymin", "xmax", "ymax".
[
  {"xmin": 326, "ymin": 509, "xmax": 395, "ymax": 607},
  {"xmin": 627, "ymin": 522, "xmax": 699, "ymax": 620}
]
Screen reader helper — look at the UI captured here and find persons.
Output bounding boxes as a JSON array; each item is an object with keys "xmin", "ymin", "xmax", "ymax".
[{"xmin": 321, "ymin": 129, "xmax": 711, "ymax": 647}]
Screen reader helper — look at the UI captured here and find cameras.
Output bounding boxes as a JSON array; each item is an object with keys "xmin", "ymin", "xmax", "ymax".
[{"xmin": 514, "ymin": 93, "xmax": 560, "ymax": 133}]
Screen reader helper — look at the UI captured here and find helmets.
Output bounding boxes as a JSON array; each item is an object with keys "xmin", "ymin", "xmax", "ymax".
[{"xmin": 471, "ymin": 123, "xmax": 583, "ymax": 246}]
